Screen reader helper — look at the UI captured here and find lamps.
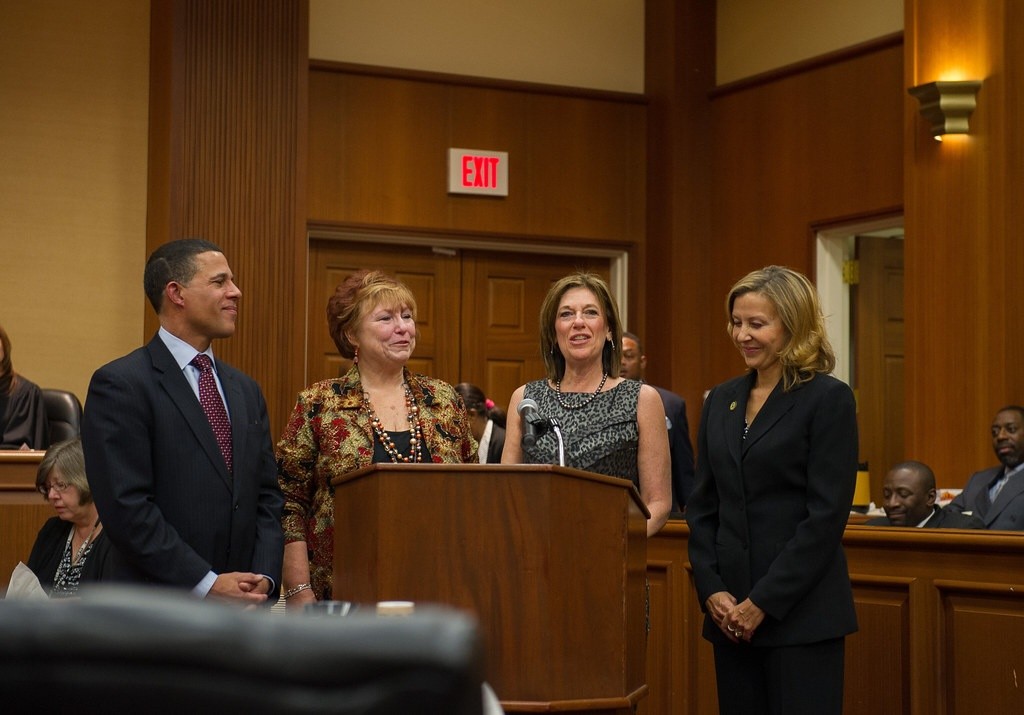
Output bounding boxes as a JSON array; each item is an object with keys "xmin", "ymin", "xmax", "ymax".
[{"xmin": 906, "ymin": 79, "xmax": 982, "ymax": 143}]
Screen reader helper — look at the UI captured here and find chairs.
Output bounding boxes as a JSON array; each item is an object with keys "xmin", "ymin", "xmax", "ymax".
[
  {"xmin": 0, "ymin": 576, "xmax": 505, "ymax": 715},
  {"xmin": 41, "ymin": 388, "xmax": 83, "ymax": 442}
]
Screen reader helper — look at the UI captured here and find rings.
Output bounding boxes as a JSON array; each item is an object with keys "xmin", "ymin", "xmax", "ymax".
[
  {"xmin": 735, "ymin": 631, "xmax": 740, "ymax": 637},
  {"xmin": 728, "ymin": 625, "xmax": 734, "ymax": 631}
]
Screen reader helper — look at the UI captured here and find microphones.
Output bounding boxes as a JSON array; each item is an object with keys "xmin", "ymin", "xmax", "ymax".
[{"xmin": 518, "ymin": 398, "xmax": 546, "ymax": 432}]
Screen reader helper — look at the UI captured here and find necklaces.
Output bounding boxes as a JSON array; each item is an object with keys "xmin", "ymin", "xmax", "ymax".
[
  {"xmin": 556, "ymin": 372, "xmax": 608, "ymax": 410},
  {"xmin": 363, "ymin": 383, "xmax": 421, "ymax": 462}
]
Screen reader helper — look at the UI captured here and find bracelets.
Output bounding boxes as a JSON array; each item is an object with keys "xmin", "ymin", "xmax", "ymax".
[{"xmin": 284, "ymin": 583, "xmax": 311, "ymax": 599}]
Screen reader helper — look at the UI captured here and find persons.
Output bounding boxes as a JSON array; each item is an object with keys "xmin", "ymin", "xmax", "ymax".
[
  {"xmin": 79, "ymin": 238, "xmax": 281, "ymax": 611},
  {"xmin": 0, "ymin": 327, "xmax": 50, "ymax": 449},
  {"xmin": 500, "ymin": 275, "xmax": 672, "ymax": 635},
  {"xmin": 864, "ymin": 461, "xmax": 987, "ymax": 529},
  {"xmin": 620, "ymin": 333, "xmax": 695, "ymax": 513},
  {"xmin": 685, "ymin": 266, "xmax": 860, "ymax": 714},
  {"xmin": 26, "ymin": 439, "xmax": 127, "ymax": 598},
  {"xmin": 944, "ymin": 404, "xmax": 1024, "ymax": 532},
  {"xmin": 452, "ymin": 383, "xmax": 505, "ymax": 463},
  {"xmin": 274, "ymin": 268, "xmax": 480, "ymax": 614}
]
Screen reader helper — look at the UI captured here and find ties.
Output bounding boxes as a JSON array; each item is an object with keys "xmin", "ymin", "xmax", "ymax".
[{"xmin": 190, "ymin": 354, "xmax": 234, "ymax": 479}]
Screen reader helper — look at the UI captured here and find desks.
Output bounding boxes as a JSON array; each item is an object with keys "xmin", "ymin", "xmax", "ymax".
[{"xmin": 0, "ymin": 449, "xmax": 59, "ymax": 594}]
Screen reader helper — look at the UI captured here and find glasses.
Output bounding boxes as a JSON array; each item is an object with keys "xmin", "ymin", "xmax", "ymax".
[{"xmin": 39, "ymin": 480, "xmax": 71, "ymax": 494}]
